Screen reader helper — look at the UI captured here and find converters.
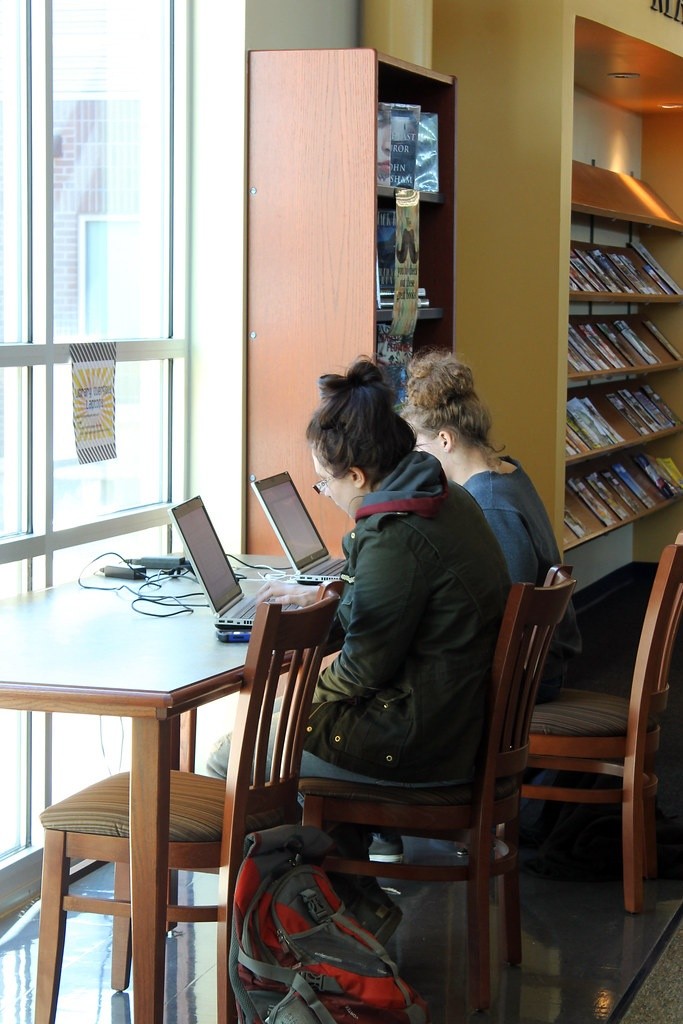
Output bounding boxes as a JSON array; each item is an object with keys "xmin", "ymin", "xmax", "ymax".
[
  {"xmin": 105, "ymin": 564, "xmax": 146, "ymax": 579},
  {"xmin": 132, "ymin": 555, "xmax": 185, "ymax": 569}
]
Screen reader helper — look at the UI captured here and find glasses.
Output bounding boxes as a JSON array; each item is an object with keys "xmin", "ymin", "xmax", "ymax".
[{"xmin": 313, "ymin": 470, "xmax": 349, "ymax": 496}]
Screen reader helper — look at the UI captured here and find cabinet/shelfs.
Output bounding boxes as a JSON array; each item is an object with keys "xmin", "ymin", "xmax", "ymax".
[
  {"xmin": 246, "ymin": 49, "xmax": 460, "ymax": 558},
  {"xmin": 433, "ymin": 0, "xmax": 683, "ymax": 563}
]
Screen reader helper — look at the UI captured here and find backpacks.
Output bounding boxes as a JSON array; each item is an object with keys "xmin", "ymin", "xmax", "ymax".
[{"xmin": 226, "ymin": 824, "xmax": 430, "ymax": 1024}]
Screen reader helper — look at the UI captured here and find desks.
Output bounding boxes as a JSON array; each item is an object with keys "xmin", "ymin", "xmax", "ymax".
[{"xmin": 0, "ymin": 550, "xmax": 347, "ymax": 1024}]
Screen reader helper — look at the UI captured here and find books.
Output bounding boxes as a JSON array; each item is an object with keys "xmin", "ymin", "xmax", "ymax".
[
  {"xmin": 376, "ymin": 209, "xmax": 430, "ymax": 309},
  {"xmin": 562, "ymin": 242, "xmax": 682, "ymax": 537},
  {"xmin": 376, "ymin": 102, "xmax": 438, "ymax": 192}
]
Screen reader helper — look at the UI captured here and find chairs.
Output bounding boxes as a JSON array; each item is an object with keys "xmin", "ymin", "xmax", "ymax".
[
  {"xmin": 297, "ymin": 565, "xmax": 578, "ymax": 1013},
  {"xmin": 520, "ymin": 529, "xmax": 683, "ymax": 914},
  {"xmin": 34, "ymin": 578, "xmax": 347, "ymax": 1024}
]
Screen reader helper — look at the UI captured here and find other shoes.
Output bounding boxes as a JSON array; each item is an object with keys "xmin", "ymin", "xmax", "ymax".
[
  {"xmin": 366, "ymin": 835, "xmax": 404, "ymax": 862},
  {"xmin": 339, "ymin": 881, "xmax": 403, "ymax": 947}
]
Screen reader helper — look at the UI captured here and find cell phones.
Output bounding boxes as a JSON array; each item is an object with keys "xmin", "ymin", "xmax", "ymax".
[{"xmin": 217, "ymin": 630, "xmax": 251, "ymax": 643}]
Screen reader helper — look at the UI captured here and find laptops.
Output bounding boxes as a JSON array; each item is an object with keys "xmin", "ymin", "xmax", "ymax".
[
  {"xmin": 251, "ymin": 471, "xmax": 347, "ymax": 585},
  {"xmin": 166, "ymin": 494, "xmax": 321, "ymax": 630}
]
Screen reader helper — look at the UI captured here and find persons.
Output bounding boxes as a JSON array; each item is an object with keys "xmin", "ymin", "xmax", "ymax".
[
  {"xmin": 368, "ymin": 346, "xmax": 581, "ymax": 864},
  {"xmin": 377, "ymin": 105, "xmax": 392, "ymax": 179},
  {"xmin": 195, "ymin": 359, "xmax": 515, "ymax": 944}
]
